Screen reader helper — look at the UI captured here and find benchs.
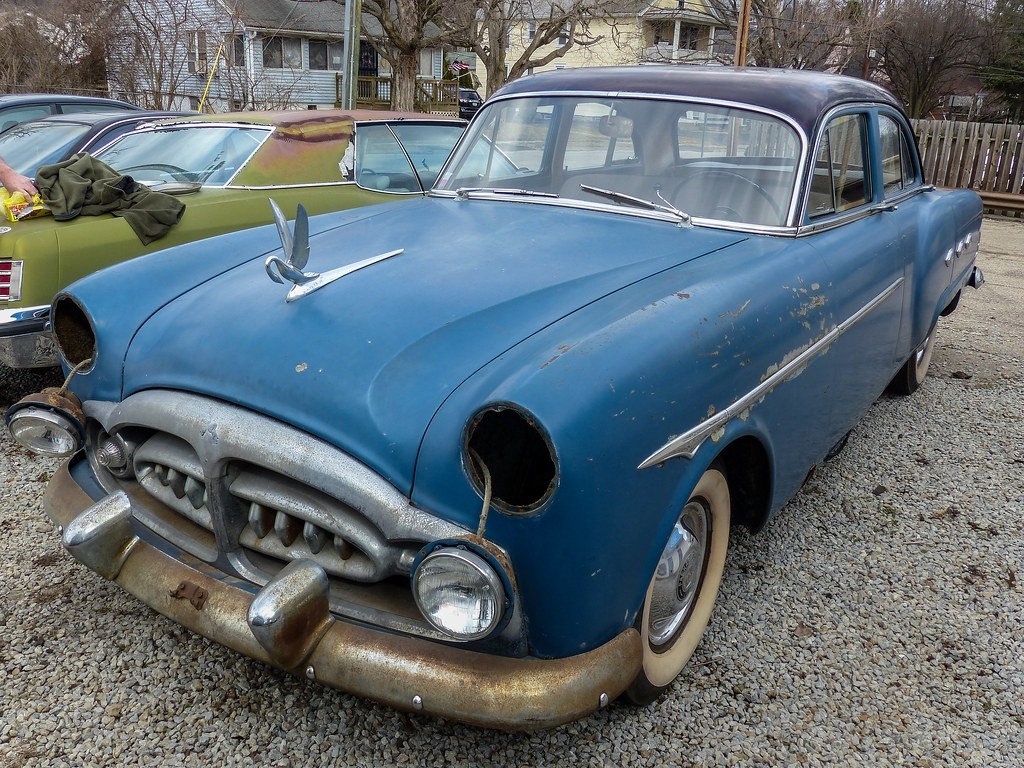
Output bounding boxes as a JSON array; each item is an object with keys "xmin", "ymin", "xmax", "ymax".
[{"xmin": 557, "ymin": 173, "xmax": 847, "ymax": 226}]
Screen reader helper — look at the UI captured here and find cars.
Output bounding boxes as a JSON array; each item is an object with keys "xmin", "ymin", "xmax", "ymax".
[
  {"xmin": 5, "ymin": 61, "xmax": 988, "ymax": 737},
  {"xmin": 4, "ymin": 109, "xmax": 535, "ymax": 367},
  {"xmin": 442, "ymin": 87, "xmax": 485, "ymax": 121},
  {"xmin": 0, "ymin": 109, "xmax": 213, "ymax": 195},
  {"xmin": 0, "ymin": 93, "xmax": 150, "ymax": 135}
]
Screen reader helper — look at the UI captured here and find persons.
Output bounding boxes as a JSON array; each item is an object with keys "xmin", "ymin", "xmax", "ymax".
[{"xmin": 0, "ymin": 157, "xmax": 37, "ymax": 206}]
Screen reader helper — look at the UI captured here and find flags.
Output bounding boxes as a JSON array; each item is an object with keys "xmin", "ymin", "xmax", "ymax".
[{"xmin": 447, "ymin": 59, "xmax": 469, "ymax": 72}]
